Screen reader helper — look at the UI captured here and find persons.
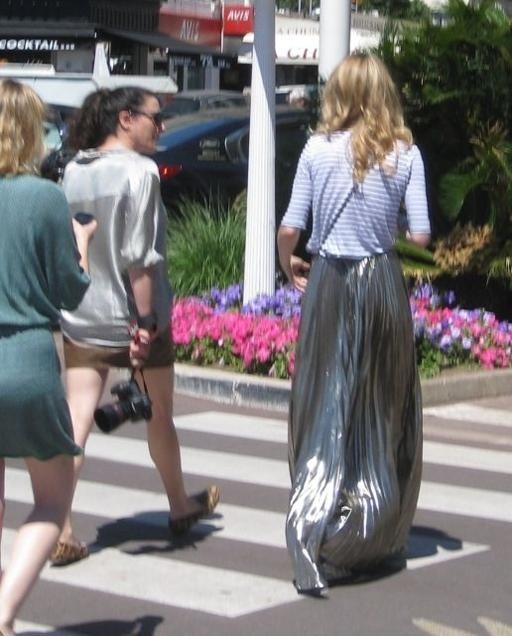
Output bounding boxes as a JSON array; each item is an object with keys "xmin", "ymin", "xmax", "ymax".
[
  {"xmin": 43, "ymin": 87, "xmax": 223, "ymax": 567},
  {"xmin": 275, "ymin": 47, "xmax": 436, "ymax": 600},
  {"xmin": 2, "ymin": 77, "xmax": 99, "ymax": 636}
]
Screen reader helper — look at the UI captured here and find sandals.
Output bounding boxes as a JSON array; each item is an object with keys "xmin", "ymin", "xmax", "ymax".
[
  {"xmin": 166, "ymin": 482, "xmax": 219, "ymax": 538},
  {"xmin": 50, "ymin": 541, "xmax": 89, "ymax": 567}
]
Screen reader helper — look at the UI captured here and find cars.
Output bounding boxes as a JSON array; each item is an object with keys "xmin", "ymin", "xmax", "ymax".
[{"xmin": 41, "ymin": 88, "xmax": 319, "ymax": 208}]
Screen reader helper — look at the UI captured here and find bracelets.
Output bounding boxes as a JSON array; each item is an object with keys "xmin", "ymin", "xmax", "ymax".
[{"xmin": 137, "ymin": 312, "xmax": 159, "ymax": 331}]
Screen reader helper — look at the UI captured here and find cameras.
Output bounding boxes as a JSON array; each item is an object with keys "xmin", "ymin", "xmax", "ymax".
[{"xmin": 94, "ymin": 378, "xmax": 153, "ymax": 433}]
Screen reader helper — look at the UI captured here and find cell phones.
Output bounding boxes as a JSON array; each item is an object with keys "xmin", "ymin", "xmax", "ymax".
[{"xmin": 74, "ymin": 210, "xmax": 96, "ymax": 228}]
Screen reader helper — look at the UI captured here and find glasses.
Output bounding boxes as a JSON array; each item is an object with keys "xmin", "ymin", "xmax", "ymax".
[{"xmin": 129, "ymin": 108, "xmax": 163, "ymax": 126}]
